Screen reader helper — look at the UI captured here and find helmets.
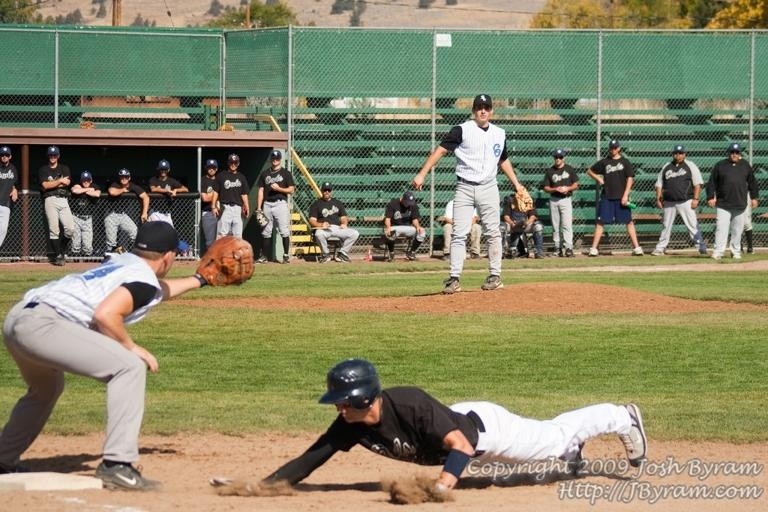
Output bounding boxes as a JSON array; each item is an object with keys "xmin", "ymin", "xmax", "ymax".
[{"xmin": 317, "ymin": 358, "xmax": 382, "ymax": 409}]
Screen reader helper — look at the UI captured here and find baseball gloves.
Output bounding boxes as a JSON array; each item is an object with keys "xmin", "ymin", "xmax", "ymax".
[
  {"xmin": 192, "ymin": 234, "xmax": 256, "ymax": 288},
  {"xmin": 513, "ymin": 190, "xmax": 538, "ymax": 217}
]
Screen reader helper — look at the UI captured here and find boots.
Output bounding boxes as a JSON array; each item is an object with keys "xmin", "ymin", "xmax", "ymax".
[
  {"xmin": 60, "ymin": 233, "xmax": 73, "ymax": 259},
  {"xmin": 49, "ymin": 238, "xmax": 65, "ymax": 266},
  {"xmin": 747, "ymin": 229, "xmax": 754, "ymax": 253}
]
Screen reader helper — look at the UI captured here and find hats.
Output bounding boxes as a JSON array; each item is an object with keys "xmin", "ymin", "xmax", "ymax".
[
  {"xmin": 402, "ymin": 191, "xmax": 416, "ymax": 207},
  {"xmin": 472, "ymin": 93, "xmax": 493, "ymax": 108},
  {"xmin": 46, "ymin": 145, "xmax": 60, "ymax": 156},
  {"xmin": 320, "ymin": 182, "xmax": 333, "ymax": 192},
  {"xmin": 671, "ymin": 145, "xmax": 687, "ymax": 154},
  {"xmin": 156, "ymin": 158, "xmax": 172, "ymax": 170},
  {"xmin": 727, "ymin": 143, "xmax": 742, "ymax": 153},
  {"xmin": 79, "ymin": 170, "xmax": 93, "ymax": 180},
  {"xmin": 204, "ymin": 158, "xmax": 218, "ymax": 169},
  {"xmin": 135, "ymin": 219, "xmax": 191, "ymax": 254},
  {"xmin": 117, "ymin": 167, "xmax": 131, "ymax": 177},
  {"xmin": 226, "ymin": 153, "xmax": 240, "ymax": 163},
  {"xmin": 0, "ymin": 147, "xmax": 12, "ymax": 157},
  {"xmin": 269, "ymin": 149, "xmax": 282, "ymax": 160},
  {"xmin": 608, "ymin": 139, "xmax": 621, "ymax": 149},
  {"xmin": 552, "ymin": 148, "xmax": 565, "ymax": 161}
]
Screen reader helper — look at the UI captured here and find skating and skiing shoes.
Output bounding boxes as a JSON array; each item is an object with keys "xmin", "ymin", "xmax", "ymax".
[
  {"xmin": 439, "ymin": 278, "xmax": 462, "ymax": 294},
  {"xmin": 481, "ymin": 276, "xmax": 505, "ymax": 291}
]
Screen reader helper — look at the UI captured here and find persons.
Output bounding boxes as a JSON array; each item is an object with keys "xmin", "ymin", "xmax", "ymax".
[
  {"xmin": 0, "ymin": 221, "xmax": 256, "ymax": 492},
  {"xmin": 215, "ymin": 153, "xmax": 250, "ymax": 236},
  {"xmin": 39, "ymin": 146, "xmax": 74, "ymax": 260},
  {"xmin": 202, "ymin": 159, "xmax": 219, "ymax": 250},
  {"xmin": 256, "ymin": 150, "xmax": 294, "ymax": 263},
  {"xmin": 0, "ymin": 147, "xmax": 17, "ymax": 247},
  {"xmin": 413, "ymin": 93, "xmax": 525, "ymax": 293},
  {"xmin": 588, "ymin": 138, "xmax": 644, "ymax": 256},
  {"xmin": 702, "ymin": 143, "xmax": 758, "ymax": 258},
  {"xmin": 384, "ymin": 192, "xmax": 425, "ymax": 262},
  {"xmin": 147, "ymin": 159, "xmax": 188, "ymax": 229},
  {"xmin": 310, "ymin": 181, "xmax": 362, "ymax": 262},
  {"xmin": 501, "ymin": 187, "xmax": 547, "ymax": 256},
  {"xmin": 740, "ymin": 190, "xmax": 753, "ymax": 253},
  {"xmin": 209, "ymin": 360, "xmax": 647, "ymax": 494},
  {"xmin": 443, "ymin": 195, "xmax": 485, "ymax": 256},
  {"xmin": 651, "ymin": 146, "xmax": 709, "ymax": 257},
  {"xmin": 70, "ymin": 170, "xmax": 101, "ymax": 257},
  {"xmin": 543, "ymin": 150, "xmax": 580, "ymax": 253},
  {"xmin": 103, "ymin": 168, "xmax": 150, "ymax": 251}
]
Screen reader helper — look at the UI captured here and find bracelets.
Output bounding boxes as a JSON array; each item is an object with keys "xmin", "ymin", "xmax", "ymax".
[{"xmin": 441, "ymin": 451, "xmax": 470, "ymax": 480}]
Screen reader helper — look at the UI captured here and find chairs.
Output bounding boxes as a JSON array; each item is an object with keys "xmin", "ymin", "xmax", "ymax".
[
  {"xmin": 381, "ymin": 217, "xmax": 415, "ymax": 262},
  {"xmin": 501, "ymin": 228, "xmax": 536, "ymax": 258},
  {"xmin": 307, "ymin": 224, "xmax": 342, "ymax": 263}
]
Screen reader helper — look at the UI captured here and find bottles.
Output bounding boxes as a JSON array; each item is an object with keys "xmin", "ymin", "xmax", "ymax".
[
  {"xmin": 188, "ymin": 245, "xmax": 193, "ymax": 257},
  {"xmin": 626, "ymin": 202, "xmax": 637, "ymax": 209},
  {"xmin": 181, "ymin": 249, "xmax": 187, "ymax": 256},
  {"xmin": 366, "ymin": 247, "xmax": 373, "ymax": 261}
]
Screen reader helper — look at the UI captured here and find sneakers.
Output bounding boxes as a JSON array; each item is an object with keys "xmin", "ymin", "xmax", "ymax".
[
  {"xmin": 632, "ymin": 248, "xmax": 644, "ymax": 256},
  {"xmin": 732, "ymin": 253, "xmax": 742, "ymax": 260},
  {"xmin": 336, "ymin": 252, "xmax": 352, "ymax": 263},
  {"xmin": 0, "ymin": 459, "xmax": 34, "ymax": 475},
  {"xmin": 651, "ymin": 249, "xmax": 665, "ymax": 257},
  {"xmin": 442, "ymin": 253, "xmax": 452, "ymax": 262},
  {"xmin": 319, "ymin": 255, "xmax": 332, "ymax": 265},
  {"xmin": 406, "ymin": 251, "xmax": 417, "ymax": 260},
  {"xmin": 616, "ymin": 401, "xmax": 650, "ymax": 468},
  {"xmin": 254, "ymin": 254, "xmax": 269, "ymax": 264},
  {"xmin": 548, "ymin": 248, "xmax": 563, "ymax": 257},
  {"xmin": 698, "ymin": 252, "xmax": 709, "ymax": 257},
  {"xmin": 565, "ymin": 248, "xmax": 574, "ymax": 257},
  {"xmin": 565, "ymin": 440, "xmax": 587, "ymax": 479},
  {"xmin": 469, "ymin": 253, "xmax": 482, "ymax": 261},
  {"xmin": 534, "ymin": 251, "xmax": 545, "ymax": 260},
  {"xmin": 710, "ymin": 254, "xmax": 724, "ymax": 261},
  {"xmin": 93, "ymin": 460, "xmax": 165, "ymax": 494},
  {"xmin": 588, "ymin": 247, "xmax": 600, "ymax": 257},
  {"xmin": 387, "ymin": 253, "xmax": 396, "ymax": 262},
  {"xmin": 282, "ymin": 254, "xmax": 292, "ymax": 265}
]
